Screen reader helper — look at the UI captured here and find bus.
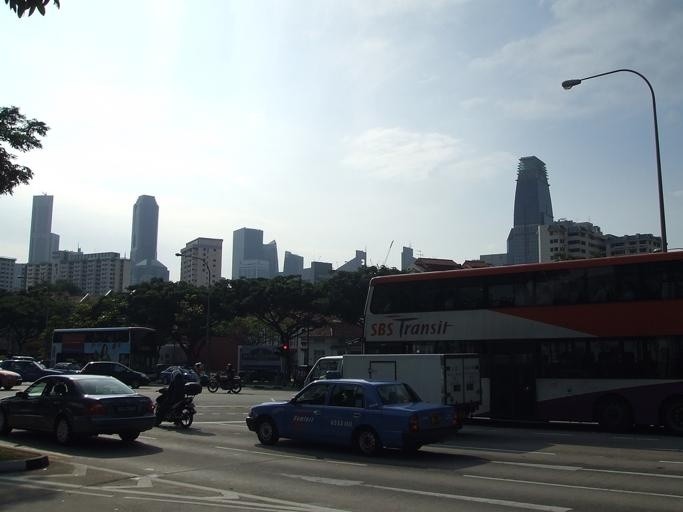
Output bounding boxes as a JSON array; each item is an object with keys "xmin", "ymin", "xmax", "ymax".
[
  {"xmin": 50, "ymin": 326, "xmax": 160, "ymax": 374},
  {"xmin": 361, "ymin": 248, "xmax": 682, "ymax": 432}
]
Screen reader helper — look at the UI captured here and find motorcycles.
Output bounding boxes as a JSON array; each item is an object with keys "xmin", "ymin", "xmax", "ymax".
[
  {"xmin": 207, "ymin": 370, "xmax": 242, "ymax": 395},
  {"xmin": 149, "ymin": 382, "xmax": 202, "ymax": 430}
]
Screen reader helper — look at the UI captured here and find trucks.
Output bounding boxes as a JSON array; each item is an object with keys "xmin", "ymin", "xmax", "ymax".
[
  {"xmin": 299, "ymin": 350, "xmax": 482, "ymax": 427},
  {"xmin": 237, "ymin": 344, "xmax": 318, "ymax": 387}
]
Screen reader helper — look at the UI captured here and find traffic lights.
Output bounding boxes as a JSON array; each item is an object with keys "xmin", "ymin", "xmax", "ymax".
[{"xmin": 279, "ymin": 334, "xmax": 290, "ymax": 353}]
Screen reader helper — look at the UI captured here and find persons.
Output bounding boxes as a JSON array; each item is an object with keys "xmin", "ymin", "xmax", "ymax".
[
  {"xmin": 119, "ymin": 354, "xmax": 129, "ymax": 366},
  {"xmin": 159, "ymin": 370, "xmax": 185, "ymax": 416},
  {"xmin": 221, "ymin": 363, "xmax": 235, "ymax": 394},
  {"xmin": 195, "ymin": 363, "xmax": 201, "ymax": 374},
  {"xmin": 89, "ymin": 343, "xmax": 97, "ymax": 353},
  {"xmin": 110, "ymin": 342, "xmax": 120, "ymax": 361},
  {"xmin": 99, "ymin": 344, "xmax": 113, "ymax": 361}
]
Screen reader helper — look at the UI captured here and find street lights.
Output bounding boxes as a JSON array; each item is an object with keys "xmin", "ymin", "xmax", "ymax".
[
  {"xmin": 560, "ymin": 69, "xmax": 667, "ymax": 252},
  {"xmin": 175, "ymin": 252, "xmax": 212, "ymax": 369},
  {"xmin": 16, "ymin": 276, "xmax": 49, "ymax": 327}
]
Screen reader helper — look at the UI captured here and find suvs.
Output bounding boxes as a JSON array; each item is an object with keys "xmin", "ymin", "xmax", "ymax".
[
  {"xmin": 79, "ymin": 361, "xmax": 150, "ymax": 389},
  {"xmin": 0, "ymin": 359, "xmax": 64, "ymax": 383}
]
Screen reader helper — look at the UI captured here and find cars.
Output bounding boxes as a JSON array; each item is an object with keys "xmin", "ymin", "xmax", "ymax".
[
  {"xmin": 48, "ymin": 362, "xmax": 83, "ymax": 376},
  {"xmin": 36, "ymin": 360, "xmax": 51, "ymax": 369},
  {"xmin": 245, "ymin": 371, "xmax": 461, "ymax": 457},
  {"xmin": 0, "ymin": 367, "xmax": 21, "ymax": 390},
  {"xmin": 0, "ymin": 373, "xmax": 155, "ymax": 447},
  {"xmin": 160, "ymin": 364, "xmax": 207, "ymax": 386},
  {"xmin": 9, "ymin": 356, "xmax": 47, "ymax": 369}
]
